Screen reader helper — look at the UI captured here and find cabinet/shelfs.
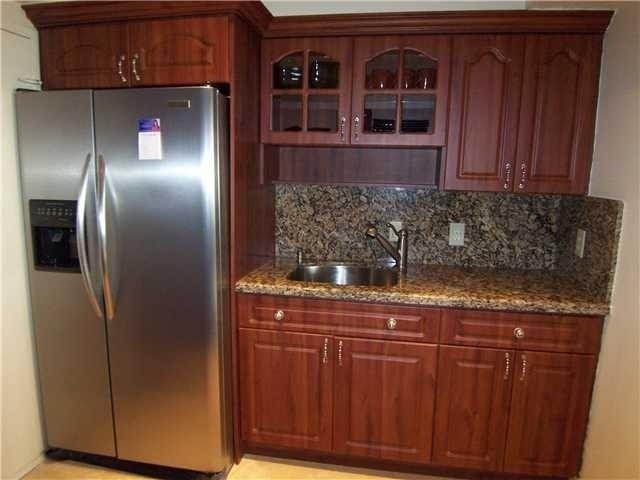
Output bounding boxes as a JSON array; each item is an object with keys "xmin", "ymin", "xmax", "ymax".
[
  {"xmin": 432, "ymin": 307, "xmax": 603, "ymax": 480},
  {"xmin": 237, "ymin": 290, "xmax": 440, "ymax": 466},
  {"xmin": 21, "ymin": 0, "xmax": 261, "ymax": 90},
  {"xmin": 259, "ymin": 0, "xmax": 451, "ymax": 146},
  {"xmin": 444, "ymin": 10, "xmax": 616, "ymax": 194}
]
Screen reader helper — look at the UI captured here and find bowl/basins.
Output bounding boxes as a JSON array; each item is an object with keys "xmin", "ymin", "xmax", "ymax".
[{"xmin": 275, "ymin": 60, "xmax": 338, "ymax": 89}]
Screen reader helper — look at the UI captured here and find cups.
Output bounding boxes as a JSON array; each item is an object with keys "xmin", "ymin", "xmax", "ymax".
[{"xmin": 370, "ymin": 68, "xmax": 435, "ymax": 91}]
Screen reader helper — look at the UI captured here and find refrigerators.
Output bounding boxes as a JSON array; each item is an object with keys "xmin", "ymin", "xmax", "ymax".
[{"xmin": 14, "ymin": 86, "xmax": 234, "ymax": 480}]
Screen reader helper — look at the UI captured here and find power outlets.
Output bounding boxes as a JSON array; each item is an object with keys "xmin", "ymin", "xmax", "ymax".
[
  {"xmin": 575, "ymin": 228, "xmax": 586, "ymax": 259},
  {"xmin": 448, "ymin": 223, "xmax": 465, "ymax": 247},
  {"xmin": 389, "ymin": 221, "xmax": 402, "ymax": 242}
]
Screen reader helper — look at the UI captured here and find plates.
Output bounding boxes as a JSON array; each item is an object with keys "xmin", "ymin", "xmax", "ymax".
[{"xmin": 373, "ymin": 118, "xmax": 429, "ymax": 132}]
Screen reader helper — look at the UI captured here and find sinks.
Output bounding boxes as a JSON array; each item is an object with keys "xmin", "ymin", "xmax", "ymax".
[{"xmin": 286, "ymin": 265, "xmax": 398, "ymax": 287}]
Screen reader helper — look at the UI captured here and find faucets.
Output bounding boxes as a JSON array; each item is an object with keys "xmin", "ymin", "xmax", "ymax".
[{"xmin": 365, "ymin": 221, "xmax": 408, "ymax": 272}]
